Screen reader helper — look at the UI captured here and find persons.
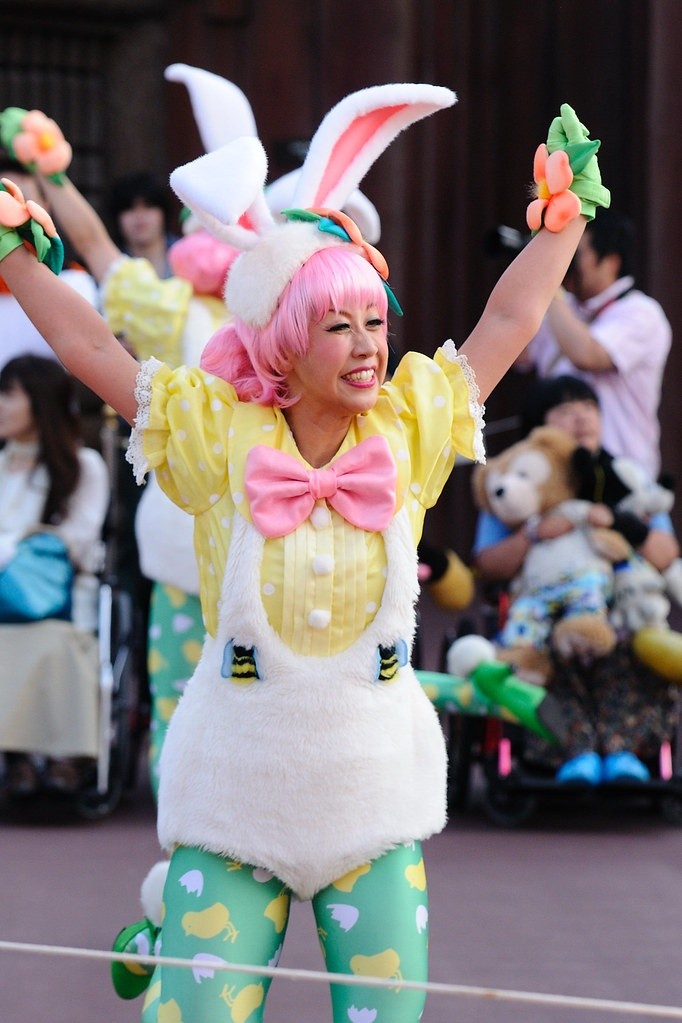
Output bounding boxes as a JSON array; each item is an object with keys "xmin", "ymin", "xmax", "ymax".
[
  {"xmin": 410, "ymin": 200, "xmax": 682, "ymax": 823},
  {"xmin": 0, "ymin": 109, "xmax": 379, "ymax": 825},
  {"xmin": 0, "ymin": 63, "xmax": 612, "ymax": 1023}
]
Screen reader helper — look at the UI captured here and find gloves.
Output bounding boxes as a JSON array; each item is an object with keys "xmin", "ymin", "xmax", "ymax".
[{"xmin": 547, "ymin": 102, "xmax": 611, "ymax": 220}]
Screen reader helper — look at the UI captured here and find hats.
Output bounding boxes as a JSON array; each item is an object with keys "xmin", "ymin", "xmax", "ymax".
[{"xmin": 521, "ymin": 369, "xmax": 599, "ymax": 436}]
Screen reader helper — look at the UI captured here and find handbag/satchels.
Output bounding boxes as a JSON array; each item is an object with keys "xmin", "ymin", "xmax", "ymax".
[{"xmin": 0, "ymin": 529, "xmax": 73, "ymax": 621}]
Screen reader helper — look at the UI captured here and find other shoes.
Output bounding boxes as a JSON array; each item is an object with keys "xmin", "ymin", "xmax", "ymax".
[
  {"xmin": 554, "ymin": 750, "xmax": 602, "ymax": 786},
  {"xmin": 604, "ymin": 750, "xmax": 650, "ymax": 784}
]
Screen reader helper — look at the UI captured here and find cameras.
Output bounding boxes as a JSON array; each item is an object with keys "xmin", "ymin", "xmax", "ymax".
[{"xmin": 507, "ymin": 229, "xmax": 574, "ymax": 277}]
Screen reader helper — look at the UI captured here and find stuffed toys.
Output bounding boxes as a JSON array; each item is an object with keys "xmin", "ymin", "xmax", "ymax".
[{"xmin": 449, "ymin": 419, "xmax": 682, "ymax": 723}]
